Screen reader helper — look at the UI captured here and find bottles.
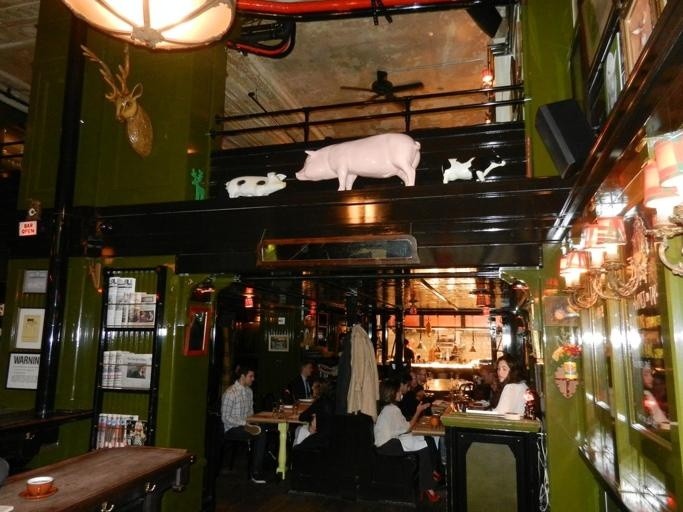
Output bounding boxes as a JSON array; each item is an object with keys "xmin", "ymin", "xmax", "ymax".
[
  {"xmin": 272, "ymin": 402, "xmax": 277, "ymax": 414},
  {"xmin": 523, "ymin": 388, "xmax": 537, "ymax": 421},
  {"xmin": 428, "ymin": 344, "xmax": 441, "ymax": 362},
  {"xmin": 461, "ymin": 401, "xmax": 465, "ymax": 413},
  {"xmin": 277, "ymin": 393, "xmax": 284, "ymax": 414},
  {"xmin": 292, "ymin": 404, "xmax": 298, "ymax": 415},
  {"xmin": 423, "ymin": 394, "xmax": 430, "ymax": 416},
  {"xmin": 448, "ymin": 373, "xmax": 459, "ymax": 390},
  {"xmin": 452, "ymin": 393, "xmax": 458, "ymax": 412},
  {"xmin": 450, "ymin": 345, "xmax": 459, "ymax": 363}
]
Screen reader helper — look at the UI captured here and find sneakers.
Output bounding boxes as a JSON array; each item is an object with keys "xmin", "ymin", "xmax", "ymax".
[
  {"xmin": 244, "ymin": 424, "xmax": 261, "ymax": 436},
  {"xmin": 251, "ymin": 473, "xmax": 266, "ymax": 484}
]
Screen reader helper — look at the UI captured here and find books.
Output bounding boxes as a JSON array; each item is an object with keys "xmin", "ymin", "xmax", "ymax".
[
  {"xmin": 94, "ymin": 411, "xmax": 148, "ymax": 451},
  {"xmin": 100, "ymin": 349, "xmax": 152, "ymax": 390},
  {"xmin": 106, "ymin": 276, "xmax": 156, "ymax": 327}
]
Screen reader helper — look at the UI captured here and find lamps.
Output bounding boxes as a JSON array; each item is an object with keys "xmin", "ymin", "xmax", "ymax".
[
  {"xmin": 559, "ymin": 130, "xmax": 683, "ymax": 310},
  {"xmin": 245, "ymin": 296, "xmax": 253, "ymax": 308},
  {"xmin": 482, "ymin": 65, "xmax": 495, "ymax": 89},
  {"xmin": 60, "ymin": 0, "xmax": 236, "ymax": 51},
  {"xmin": 417, "ymin": 313, "xmax": 476, "ymax": 354}
]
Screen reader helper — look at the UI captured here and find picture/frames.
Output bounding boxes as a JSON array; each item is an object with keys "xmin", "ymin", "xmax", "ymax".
[
  {"xmin": 268, "ymin": 334, "xmax": 289, "ymax": 352},
  {"xmin": 182, "ymin": 305, "xmax": 211, "ymax": 357}
]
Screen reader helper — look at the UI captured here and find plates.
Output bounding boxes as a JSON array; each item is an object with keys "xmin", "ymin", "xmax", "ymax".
[
  {"xmin": 468, "ymin": 400, "xmax": 490, "ymax": 410},
  {"xmin": 17, "ymin": 484, "xmax": 58, "ymax": 500}
]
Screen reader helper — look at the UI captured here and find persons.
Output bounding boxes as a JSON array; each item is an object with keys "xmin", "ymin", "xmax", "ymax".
[
  {"xmin": 0, "ymin": 458, "xmax": 11, "ymax": 488},
  {"xmin": 221, "ymin": 365, "xmax": 273, "ymax": 484},
  {"xmin": 479, "ymin": 355, "xmax": 534, "ymax": 417},
  {"xmin": 276, "ymin": 342, "xmax": 350, "ymax": 482},
  {"xmin": 639, "ymin": 363, "xmax": 673, "ymax": 429},
  {"xmin": 373, "ymin": 366, "xmax": 444, "ymax": 501}
]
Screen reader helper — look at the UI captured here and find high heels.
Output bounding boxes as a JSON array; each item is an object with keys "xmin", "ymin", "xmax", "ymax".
[{"xmin": 419, "ymin": 490, "xmax": 439, "ymax": 503}]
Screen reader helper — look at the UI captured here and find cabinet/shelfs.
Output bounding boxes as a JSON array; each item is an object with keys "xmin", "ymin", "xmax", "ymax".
[{"xmin": 89, "ymin": 266, "xmax": 162, "ymax": 451}]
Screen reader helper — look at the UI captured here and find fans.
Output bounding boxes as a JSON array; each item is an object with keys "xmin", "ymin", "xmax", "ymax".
[{"xmin": 340, "ymin": 70, "xmax": 423, "ymax": 109}]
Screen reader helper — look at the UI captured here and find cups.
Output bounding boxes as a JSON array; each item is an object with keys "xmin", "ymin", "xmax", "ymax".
[
  {"xmin": 430, "ymin": 414, "xmax": 439, "ymax": 427},
  {"xmin": 26, "ymin": 476, "xmax": 54, "ymax": 494},
  {"xmin": 563, "ymin": 361, "xmax": 576, "ymax": 377}
]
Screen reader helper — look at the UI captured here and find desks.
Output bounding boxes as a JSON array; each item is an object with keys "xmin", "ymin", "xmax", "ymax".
[{"xmin": 0, "ymin": 446, "xmax": 198, "ymax": 512}]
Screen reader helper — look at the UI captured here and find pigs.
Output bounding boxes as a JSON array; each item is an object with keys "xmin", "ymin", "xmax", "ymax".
[
  {"xmin": 441, "ymin": 153, "xmax": 507, "ymax": 184},
  {"xmin": 295, "ymin": 132, "xmax": 421, "ymax": 191},
  {"xmin": 225, "ymin": 172, "xmax": 287, "ymax": 198}
]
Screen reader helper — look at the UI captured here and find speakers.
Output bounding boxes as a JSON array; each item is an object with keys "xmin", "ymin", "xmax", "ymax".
[
  {"xmin": 467, "ymin": 0, "xmax": 503, "ymax": 41},
  {"xmin": 534, "ymin": 98, "xmax": 597, "ymax": 182}
]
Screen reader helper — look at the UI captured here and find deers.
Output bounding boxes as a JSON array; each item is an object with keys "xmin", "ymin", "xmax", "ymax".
[{"xmin": 80, "ymin": 41, "xmax": 154, "ymax": 161}]
